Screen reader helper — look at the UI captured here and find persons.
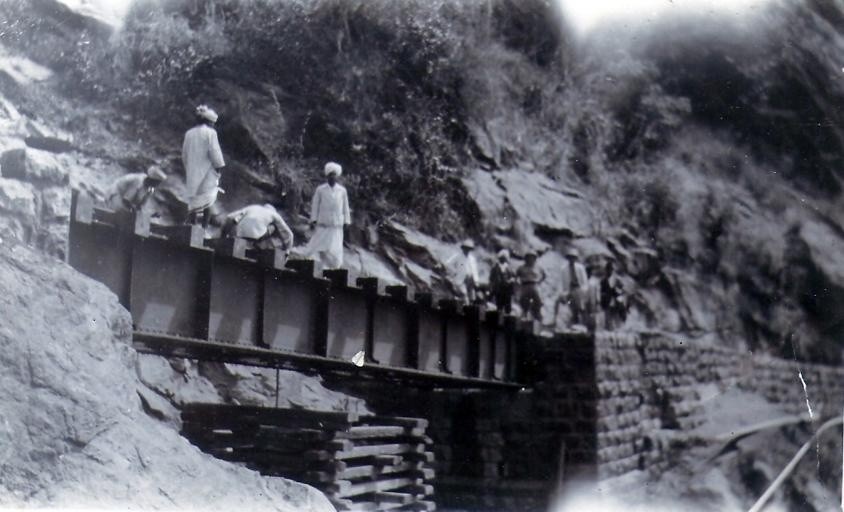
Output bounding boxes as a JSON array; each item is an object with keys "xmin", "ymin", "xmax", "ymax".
[
  {"xmin": 517, "ymin": 251, "xmax": 549, "ymax": 322},
  {"xmin": 180, "ymin": 104, "xmax": 227, "ymax": 230},
  {"xmin": 552, "ymin": 248, "xmax": 626, "ymax": 331},
  {"xmin": 445, "ymin": 240, "xmax": 481, "ymax": 307},
  {"xmin": 488, "ymin": 248, "xmax": 517, "ymax": 314},
  {"xmin": 297, "ymin": 160, "xmax": 353, "ymax": 269},
  {"xmin": 102, "ymin": 166, "xmax": 167, "ymax": 215},
  {"xmin": 225, "ymin": 198, "xmax": 294, "ymax": 258}
]
